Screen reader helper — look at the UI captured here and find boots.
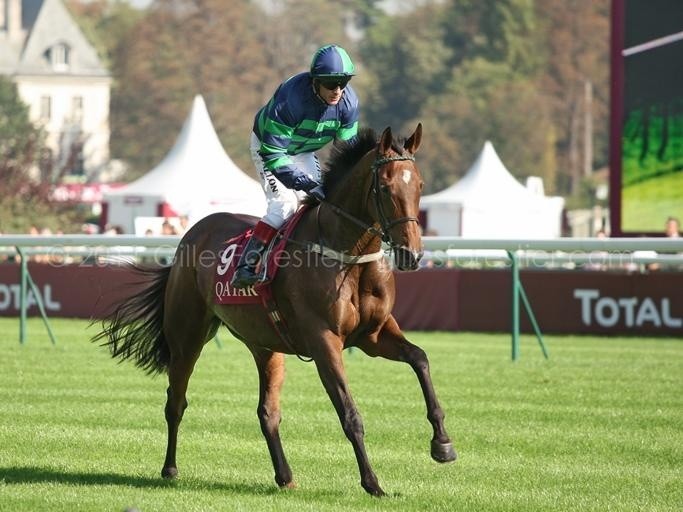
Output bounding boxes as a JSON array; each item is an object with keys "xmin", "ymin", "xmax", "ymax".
[{"xmin": 231, "ymin": 221, "xmax": 278, "ymax": 288}]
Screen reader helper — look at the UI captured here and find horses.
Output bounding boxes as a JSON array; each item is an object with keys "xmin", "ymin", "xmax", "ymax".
[{"xmin": 85, "ymin": 120, "xmax": 457, "ymax": 500}]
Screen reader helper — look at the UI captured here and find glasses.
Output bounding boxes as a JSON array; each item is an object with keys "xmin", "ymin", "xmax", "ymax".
[{"xmin": 316, "ymin": 77, "xmax": 351, "ymax": 90}]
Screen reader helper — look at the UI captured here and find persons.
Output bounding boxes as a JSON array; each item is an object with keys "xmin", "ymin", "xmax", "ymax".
[
  {"xmin": 229, "ymin": 43, "xmax": 359, "ymax": 291},
  {"xmin": 0, "ymin": 217, "xmax": 179, "ymax": 268},
  {"xmin": 569, "ymin": 216, "xmax": 682, "ymax": 271}
]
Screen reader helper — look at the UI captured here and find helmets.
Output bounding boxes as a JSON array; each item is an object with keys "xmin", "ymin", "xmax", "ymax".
[{"xmin": 310, "ymin": 44, "xmax": 356, "ymax": 76}]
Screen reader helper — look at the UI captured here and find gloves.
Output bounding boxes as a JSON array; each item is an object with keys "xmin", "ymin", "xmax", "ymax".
[{"xmin": 304, "ymin": 181, "xmax": 325, "ymax": 200}]
